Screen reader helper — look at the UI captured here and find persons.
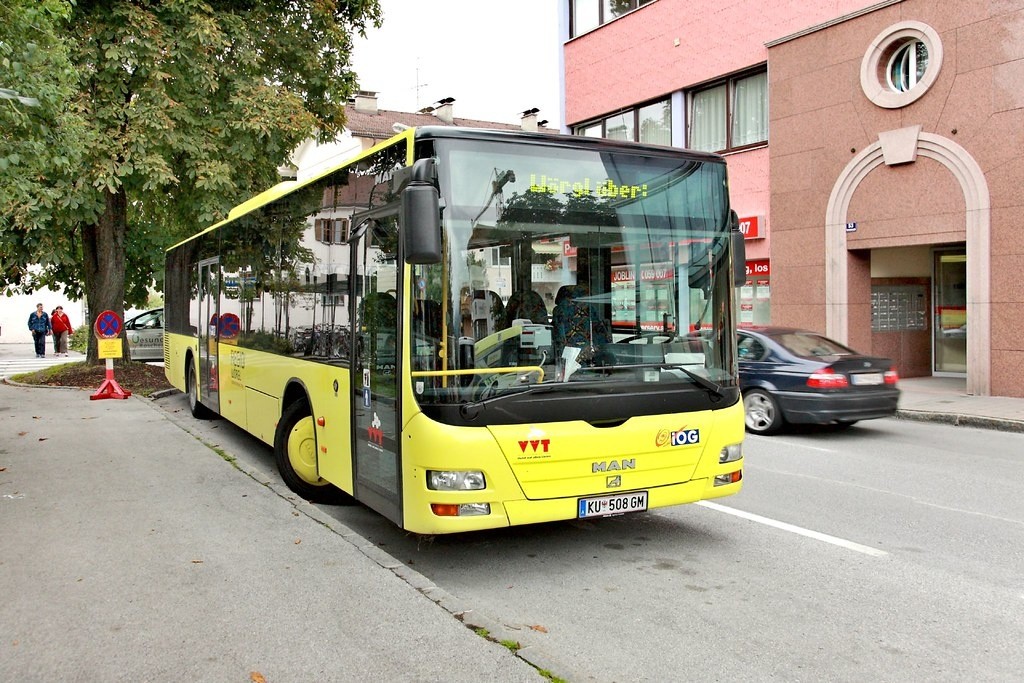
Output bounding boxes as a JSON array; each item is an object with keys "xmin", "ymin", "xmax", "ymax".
[
  {"xmin": 28, "ymin": 303, "xmax": 53, "ymax": 358},
  {"xmin": 50, "ymin": 305, "xmax": 74, "ymax": 357}
]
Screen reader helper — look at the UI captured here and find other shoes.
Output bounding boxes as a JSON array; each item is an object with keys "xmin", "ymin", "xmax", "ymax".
[
  {"xmin": 36, "ymin": 354, "xmax": 39, "ymax": 357},
  {"xmin": 64, "ymin": 352, "xmax": 68, "ymax": 356},
  {"xmin": 53, "ymin": 353, "xmax": 59, "ymax": 356},
  {"xmin": 41, "ymin": 354, "xmax": 45, "ymax": 358}
]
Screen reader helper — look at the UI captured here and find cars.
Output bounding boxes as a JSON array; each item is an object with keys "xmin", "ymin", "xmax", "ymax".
[
  {"xmin": 669, "ymin": 326, "xmax": 901, "ymax": 437},
  {"xmin": 120, "ymin": 306, "xmax": 164, "ymax": 363}
]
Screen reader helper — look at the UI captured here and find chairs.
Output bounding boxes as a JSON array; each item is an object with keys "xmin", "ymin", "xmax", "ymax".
[{"xmin": 356, "ymin": 283, "xmax": 614, "ymax": 388}]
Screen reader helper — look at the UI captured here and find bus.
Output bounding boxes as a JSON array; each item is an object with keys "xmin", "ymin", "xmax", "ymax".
[{"xmin": 163, "ymin": 121, "xmax": 747, "ymax": 536}]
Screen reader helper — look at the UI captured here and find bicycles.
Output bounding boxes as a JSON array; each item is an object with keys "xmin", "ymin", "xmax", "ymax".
[{"xmin": 283, "ymin": 323, "xmax": 366, "ymax": 360}]
